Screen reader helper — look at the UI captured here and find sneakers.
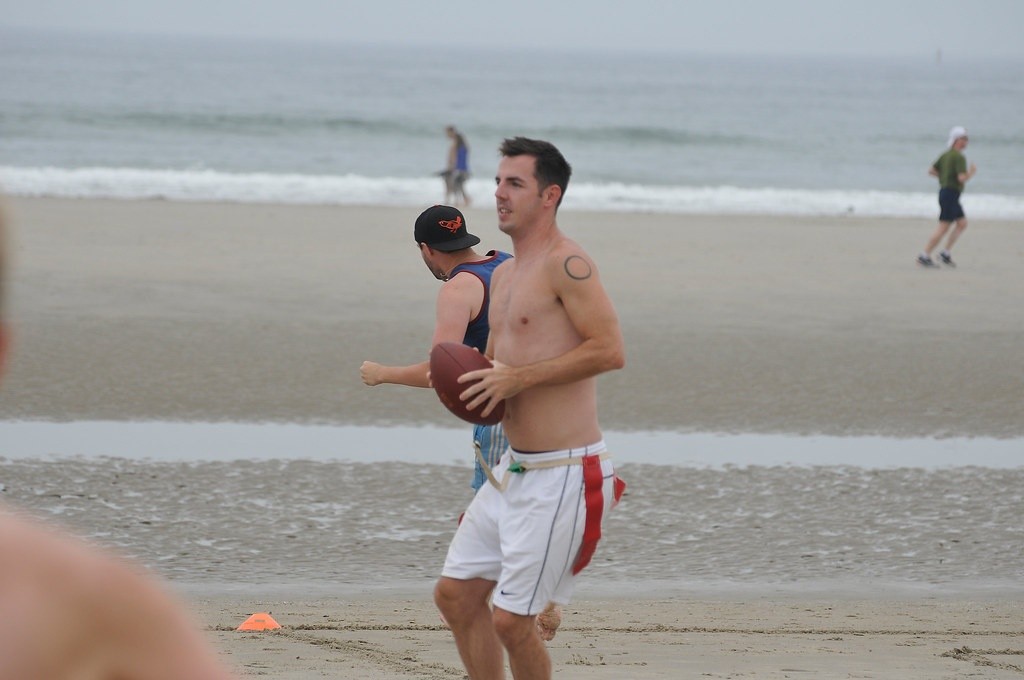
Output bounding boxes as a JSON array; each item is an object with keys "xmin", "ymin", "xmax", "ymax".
[
  {"xmin": 914, "ymin": 255, "xmax": 940, "ymax": 269},
  {"xmin": 936, "ymin": 251, "xmax": 956, "ymax": 269}
]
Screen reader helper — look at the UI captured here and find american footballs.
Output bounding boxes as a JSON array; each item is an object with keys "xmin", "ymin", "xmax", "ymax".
[{"xmin": 428, "ymin": 340, "xmax": 507, "ymax": 428}]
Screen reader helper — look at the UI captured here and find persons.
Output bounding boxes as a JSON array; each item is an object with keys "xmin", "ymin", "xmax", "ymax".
[
  {"xmin": 916, "ymin": 126, "xmax": 977, "ymax": 267},
  {"xmin": 360, "ymin": 205, "xmax": 562, "ymax": 641},
  {"xmin": 427, "ymin": 137, "xmax": 626, "ymax": 680},
  {"xmin": 440, "ymin": 127, "xmax": 471, "ymax": 205},
  {"xmin": 1, "ymin": 206, "xmax": 242, "ymax": 679}
]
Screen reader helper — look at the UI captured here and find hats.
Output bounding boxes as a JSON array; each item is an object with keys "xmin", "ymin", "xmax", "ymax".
[
  {"xmin": 945, "ymin": 125, "xmax": 970, "ymax": 148},
  {"xmin": 414, "ymin": 204, "xmax": 481, "ymax": 252}
]
